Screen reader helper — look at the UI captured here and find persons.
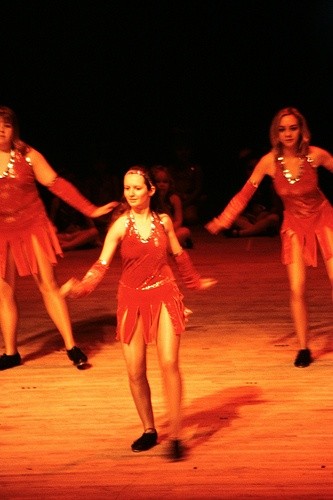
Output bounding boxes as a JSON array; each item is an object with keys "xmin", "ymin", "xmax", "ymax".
[
  {"xmin": 0, "ymin": 106, "xmax": 119, "ymax": 372},
  {"xmin": 151, "ymin": 166, "xmax": 192, "ymax": 249},
  {"xmin": 60, "ymin": 165, "xmax": 218, "ymax": 462},
  {"xmin": 225, "ymin": 179, "xmax": 279, "ymax": 237},
  {"xmin": 47, "ymin": 182, "xmax": 102, "ymax": 251},
  {"xmin": 205, "ymin": 108, "xmax": 333, "ymax": 369}
]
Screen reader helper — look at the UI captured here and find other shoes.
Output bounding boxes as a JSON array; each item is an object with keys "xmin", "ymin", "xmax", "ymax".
[
  {"xmin": 130, "ymin": 427, "xmax": 158, "ymax": 451},
  {"xmin": 66, "ymin": 346, "xmax": 87, "ymax": 367},
  {"xmin": 0, "ymin": 352, "xmax": 23, "ymax": 371},
  {"xmin": 294, "ymin": 348, "xmax": 312, "ymax": 370},
  {"xmin": 167, "ymin": 439, "xmax": 188, "ymax": 463}
]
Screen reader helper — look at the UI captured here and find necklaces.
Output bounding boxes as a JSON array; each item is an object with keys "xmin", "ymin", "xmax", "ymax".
[
  {"xmin": 0, "ymin": 151, "xmax": 16, "ymax": 179},
  {"xmin": 276, "ymin": 152, "xmax": 306, "ymax": 184},
  {"xmin": 129, "ymin": 211, "xmax": 157, "ymax": 242}
]
